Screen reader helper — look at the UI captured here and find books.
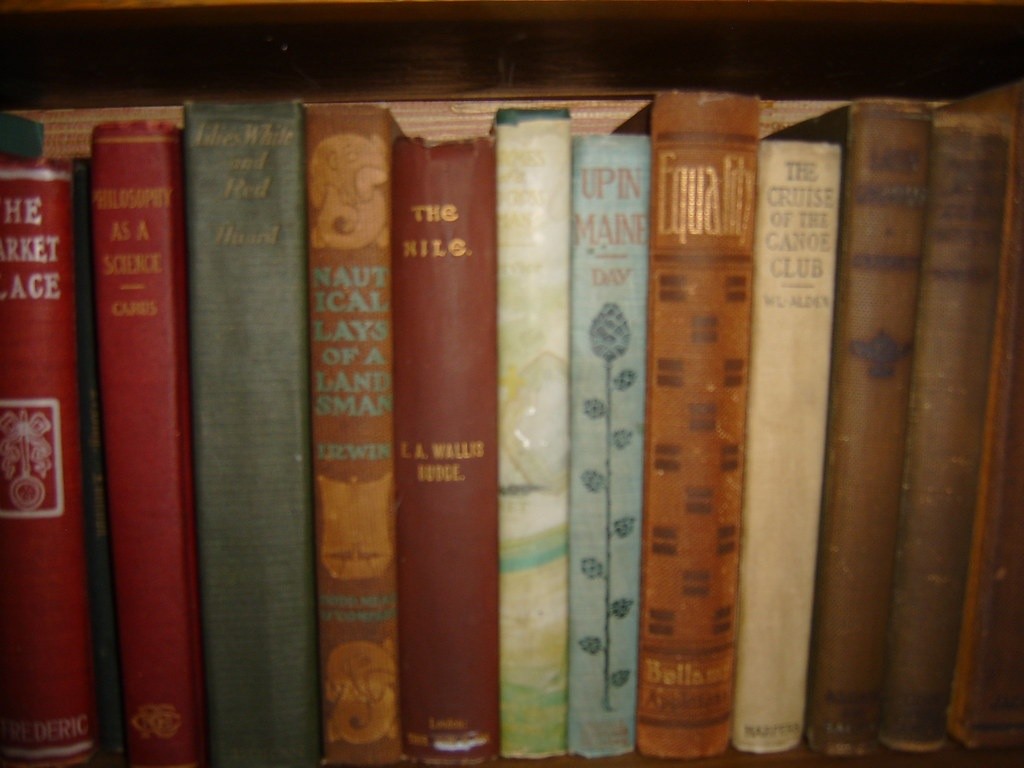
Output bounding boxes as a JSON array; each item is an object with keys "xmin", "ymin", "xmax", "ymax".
[
  {"xmin": 759, "ymin": 99, "xmax": 951, "ymax": 755},
  {"xmin": 0, "ymin": 99, "xmax": 651, "ymax": 768},
  {"xmin": 729, "ymin": 139, "xmax": 841, "ymax": 752},
  {"xmin": 876, "ymin": 112, "xmax": 1012, "ymax": 751},
  {"xmin": 609, "ymin": 90, "xmax": 759, "ymax": 756}
]
